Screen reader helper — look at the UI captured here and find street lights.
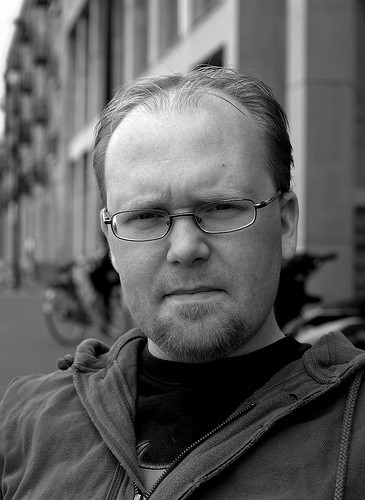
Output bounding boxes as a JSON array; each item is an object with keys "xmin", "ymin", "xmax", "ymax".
[{"xmin": 4, "ymin": 65, "xmax": 22, "ymax": 289}]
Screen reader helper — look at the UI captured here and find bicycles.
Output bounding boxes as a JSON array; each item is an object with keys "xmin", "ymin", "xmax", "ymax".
[{"xmin": 42, "ymin": 250, "xmax": 134, "ymax": 349}]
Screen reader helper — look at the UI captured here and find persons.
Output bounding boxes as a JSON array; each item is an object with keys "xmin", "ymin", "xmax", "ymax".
[{"xmin": 0, "ymin": 65, "xmax": 364, "ymax": 500}]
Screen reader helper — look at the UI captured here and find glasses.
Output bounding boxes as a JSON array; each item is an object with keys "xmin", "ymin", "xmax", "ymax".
[{"xmin": 102, "ymin": 188, "xmax": 291, "ymax": 243}]
